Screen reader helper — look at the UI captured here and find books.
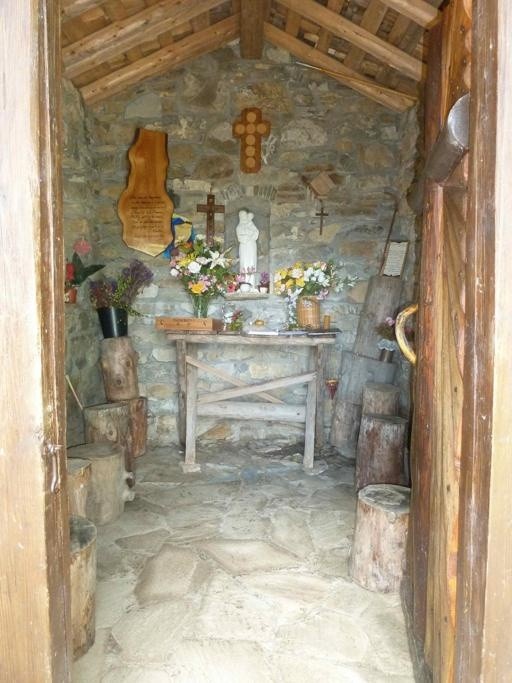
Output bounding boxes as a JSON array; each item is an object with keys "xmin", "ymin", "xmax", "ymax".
[
  {"xmin": 307, "ymin": 327, "xmax": 343, "ymax": 336},
  {"xmin": 244, "ymin": 323, "xmax": 278, "ymax": 336}
]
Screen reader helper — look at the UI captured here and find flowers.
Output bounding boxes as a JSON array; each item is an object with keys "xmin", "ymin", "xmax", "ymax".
[
  {"xmin": 85, "ymin": 256, "xmax": 156, "ymax": 318},
  {"xmin": 260, "ymin": 272, "xmax": 270, "ymax": 286},
  {"xmin": 371, "ymin": 316, "xmax": 414, "ymax": 342},
  {"xmin": 273, "ymin": 257, "xmax": 359, "ymax": 331},
  {"xmin": 65, "ymin": 238, "xmax": 107, "ymax": 288},
  {"xmin": 169, "ymin": 234, "xmax": 256, "ymax": 318}
]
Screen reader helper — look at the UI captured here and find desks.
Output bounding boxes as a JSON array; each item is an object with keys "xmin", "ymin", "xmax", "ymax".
[{"xmin": 165, "ymin": 331, "xmax": 336, "ymax": 468}]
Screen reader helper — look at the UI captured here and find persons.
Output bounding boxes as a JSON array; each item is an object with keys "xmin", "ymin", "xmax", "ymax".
[{"xmin": 235, "ymin": 209, "xmax": 260, "ymax": 273}]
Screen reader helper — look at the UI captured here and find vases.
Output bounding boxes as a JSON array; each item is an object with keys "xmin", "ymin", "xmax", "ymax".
[
  {"xmin": 259, "ymin": 287, "xmax": 268, "ymax": 293},
  {"xmin": 97, "ymin": 309, "xmax": 129, "ymax": 339},
  {"xmin": 381, "ymin": 338, "xmax": 395, "ymax": 362},
  {"xmin": 296, "ymin": 296, "xmax": 321, "ymax": 331},
  {"xmin": 64, "ymin": 286, "xmax": 77, "ymax": 303},
  {"xmin": 189, "ymin": 294, "xmax": 211, "ymax": 319}
]
